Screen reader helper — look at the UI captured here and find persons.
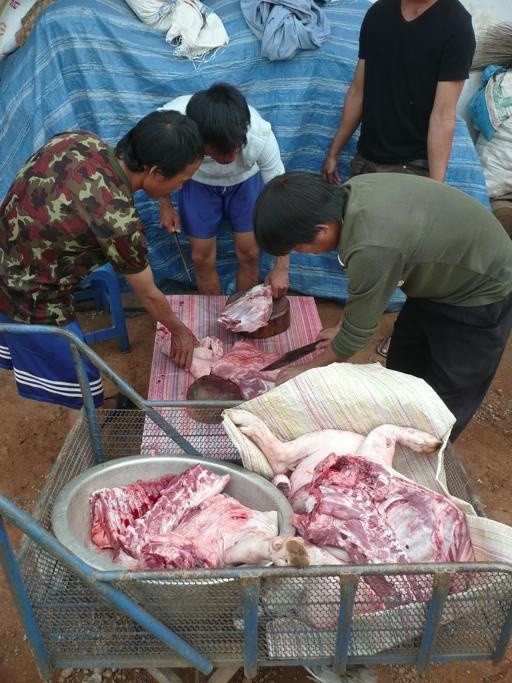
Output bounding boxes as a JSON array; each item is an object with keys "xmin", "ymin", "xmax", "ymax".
[
  {"xmin": 153, "ymin": 81, "xmax": 294, "ymax": 301},
  {"xmin": 252, "ymin": 167, "xmax": 512, "ymax": 443},
  {"xmin": 320, "ymin": 0, "xmax": 476, "ymax": 185},
  {"xmin": 0, "ymin": 108, "xmax": 207, "ymax": 412}
]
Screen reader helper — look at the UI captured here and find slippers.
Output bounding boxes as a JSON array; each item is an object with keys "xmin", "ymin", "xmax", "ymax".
[{"xmin": 98, "ymin": 388, "xmax": 131, "ymax": 429}]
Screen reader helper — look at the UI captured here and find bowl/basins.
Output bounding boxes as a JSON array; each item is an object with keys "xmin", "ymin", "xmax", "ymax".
[{"xmin": 50, "ymin": 453, "xmax": 296, "ymax": 618}]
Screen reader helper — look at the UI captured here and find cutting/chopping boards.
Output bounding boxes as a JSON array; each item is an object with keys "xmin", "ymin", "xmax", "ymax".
[
  {"xmin": 185, "ymin": 374, "xmax": 242, "ymax": 425},
  {"xmin": 225, "ymin": 288, "xmax": 291, "ymax": 339}
]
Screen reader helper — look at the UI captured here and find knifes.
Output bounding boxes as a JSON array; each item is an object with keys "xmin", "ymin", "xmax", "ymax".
[
  {"xmin": 172, "ymin": 226, "xmax": 193, "ymax": 283},
  {"xmin": 259, "ymin": 338, "xmax": 324, "ymax": 373}
]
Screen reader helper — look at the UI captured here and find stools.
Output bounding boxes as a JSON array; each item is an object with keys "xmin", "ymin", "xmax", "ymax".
[{"xmin": 64, "ymin": 261, "xmax": 133, "ymax": 353}]
